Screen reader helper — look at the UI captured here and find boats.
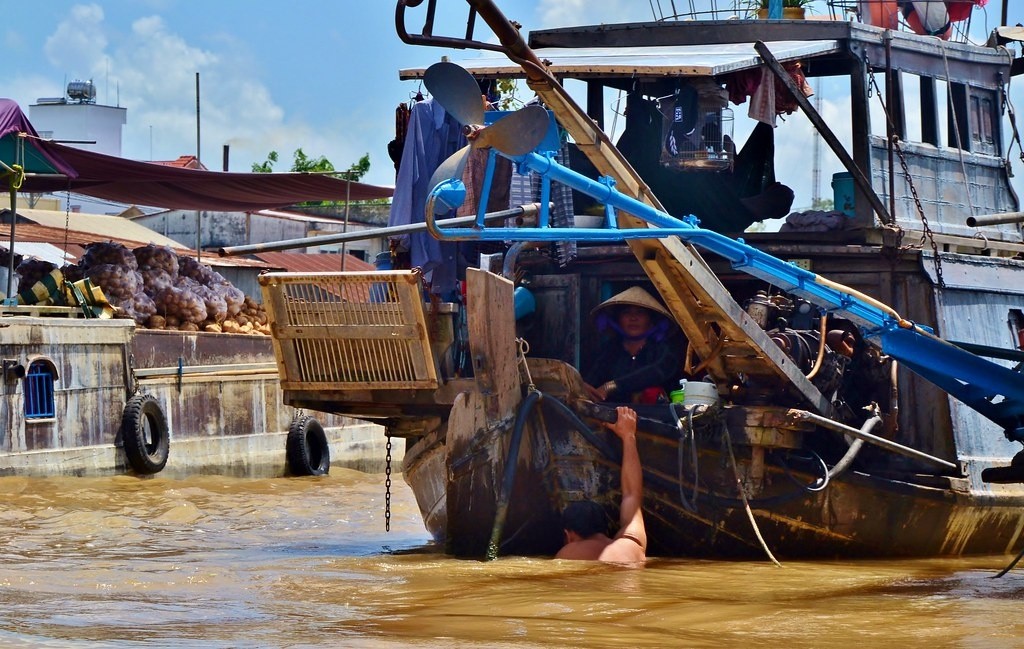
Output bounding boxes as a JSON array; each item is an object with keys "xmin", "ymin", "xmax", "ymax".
[
  {"xmin": 256, "ymin": 0, "xmax": 1024, "ymax": 565},
  {"xmin": 0, "ymin": 99, "xmax": 418, "ymax": 474}
]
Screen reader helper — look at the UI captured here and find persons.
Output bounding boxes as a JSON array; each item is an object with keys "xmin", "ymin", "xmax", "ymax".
[
  {"xmin": 556, "ymin": 406, "xmax": 647, "ymax": 562},
  {"xmin": 582, "ymin": 286, "xmax": 690, "ymax": 405}
]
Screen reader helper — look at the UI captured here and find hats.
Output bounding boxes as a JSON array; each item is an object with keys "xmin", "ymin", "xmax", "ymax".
[{"xmin": 590, "ymin": 286, "xmax": 677, "ymax": 330}]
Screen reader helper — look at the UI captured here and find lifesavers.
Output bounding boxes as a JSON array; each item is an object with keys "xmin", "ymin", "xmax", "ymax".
[
  {"xmin": 122, "ymin": 394, "xmax": 170, "ymax": 476},
  {"xmin": 286, "ymin": 415, "xmax": 330, "ymax": 477}
]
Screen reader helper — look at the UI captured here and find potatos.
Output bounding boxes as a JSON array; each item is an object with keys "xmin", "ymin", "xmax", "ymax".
[
  {"xmin": 78, "ymin": 241, "xmax": 272, "ymax": 336},
  {"xmin": 0, "ymin": 245, "xmax": 87, "ymax": 291}
]
